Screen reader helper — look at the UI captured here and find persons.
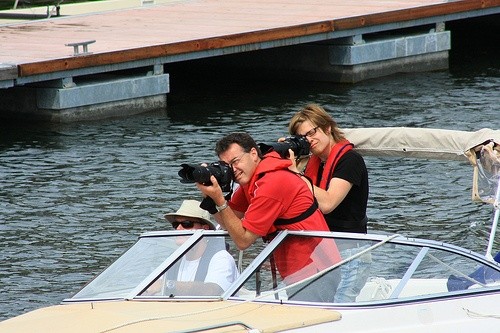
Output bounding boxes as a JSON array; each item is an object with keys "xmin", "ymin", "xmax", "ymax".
[
  {"xmin": 140, "ymin": 200, "xmax": 239, "ymax": 297},
  {"xmin": 277, "ymin": 104, "xmax": 372, "ymax": 303},
  {"xmin": 196, "ymin": 133, "xmax": 342, "ymax": 303}
]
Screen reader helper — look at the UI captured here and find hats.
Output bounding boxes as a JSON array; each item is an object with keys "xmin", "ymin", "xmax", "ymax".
[{"xmin": 164, "ymin": 199, "xmax": 216, "ymax": 230}]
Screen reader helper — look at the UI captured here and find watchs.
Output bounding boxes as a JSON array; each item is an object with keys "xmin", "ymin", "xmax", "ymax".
[
  {"xmin": 164, "ymin": 279, "xmax": 176, "ymax": 293},
  {"xmin": 216, "ymin": 199, "xmax": 228, "ymax": 212}
]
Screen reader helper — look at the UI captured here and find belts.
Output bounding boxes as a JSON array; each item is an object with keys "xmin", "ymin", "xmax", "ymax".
[{"xmin": 337, "ymin": 241, "xmax": 367, "ymax": 252}]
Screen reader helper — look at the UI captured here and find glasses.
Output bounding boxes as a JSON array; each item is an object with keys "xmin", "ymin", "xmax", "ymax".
[
  {"xmin": 229, "ymin": 152, "xmax": 246, "ymax": 168},
  {"xmin": 172, "ymin": 220, "xmax": 200, "ymax": 229},
  {"xmin": 301, "ymin": 126, "xmax": 320, "ymax": 141}
]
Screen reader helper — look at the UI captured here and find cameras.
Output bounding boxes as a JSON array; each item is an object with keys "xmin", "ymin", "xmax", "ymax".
[
  {"xmin": 178, "ymin": 161, "xmax": 231, "ymax": 185},
  {"xmin": 257, "ymin": 135, "xmax": 310, "ymax": 158}
]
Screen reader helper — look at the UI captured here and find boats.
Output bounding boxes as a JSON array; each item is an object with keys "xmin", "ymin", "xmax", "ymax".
[{"xmin": 0, "ymin": 127, "xmax": 500, "ymax": 331}]
